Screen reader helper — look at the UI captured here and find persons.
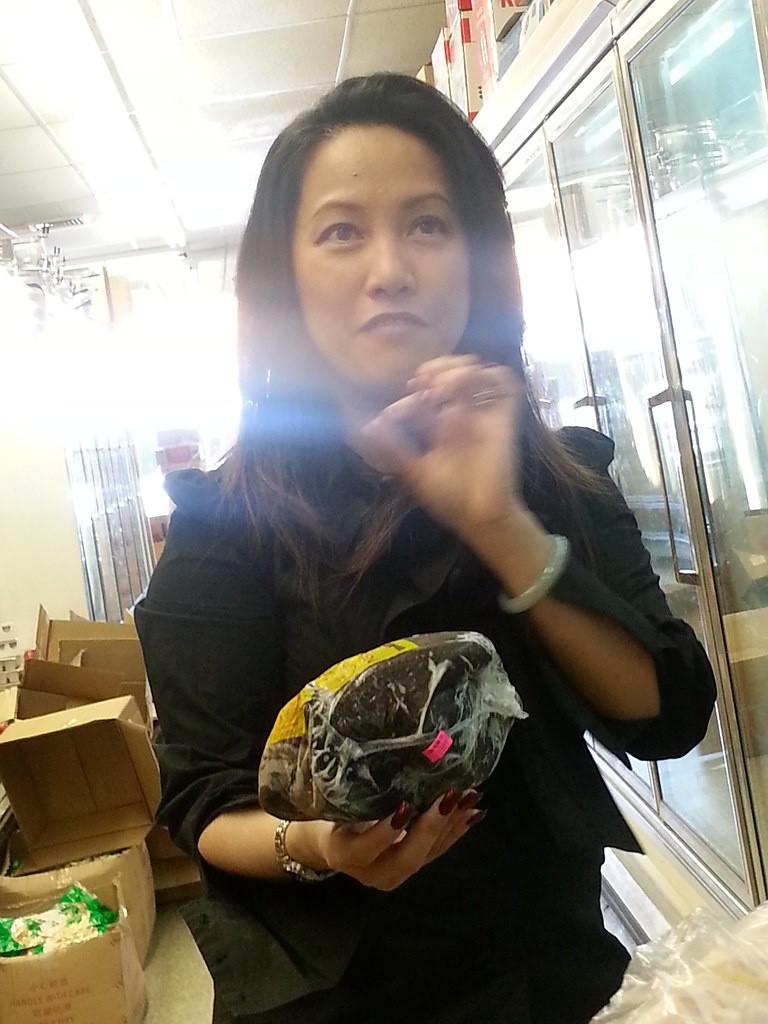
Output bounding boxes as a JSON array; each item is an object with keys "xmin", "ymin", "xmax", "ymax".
[{"xmin": 133, "ymin": 71, "xmax": 718, "ymax": 1024}]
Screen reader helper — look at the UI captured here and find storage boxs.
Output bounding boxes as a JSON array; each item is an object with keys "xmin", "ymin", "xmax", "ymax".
[
  {"xmin": 415, "ymin": 0, "xmax": 554, "ymax": 122},
  {"xmin": 14, "ymin": 656, "xmax": 125, "ymax": 720},
  {"xmin": 0, "ymin": 839, "xmax": 156, "ymax": 969},
  {"xmin": 33, "ymin": 603, "xmax": 148, "ymax": 723},
  {"xmin": 723, "ymin": 608, "xmax": 768, "ymax": 759},
  {"xmin": 722, "ymin": 515, "xmax": 768, "ymax": 610},
  {"xmin": 0, "ymin": 694, "xmax": 162, "ymax": 877},
  {"xmin": 0, "ymin": 873, "xmax": 151, "ymax": 1024}
]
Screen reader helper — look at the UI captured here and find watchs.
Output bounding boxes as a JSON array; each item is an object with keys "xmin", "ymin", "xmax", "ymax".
[{"xmin": 274, "ymin": 819, "xmax": 338, "ymax": 884}]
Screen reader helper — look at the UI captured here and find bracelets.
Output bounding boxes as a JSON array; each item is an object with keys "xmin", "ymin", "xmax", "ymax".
[{"xmin": 497, "ymin": 534, "xmax": 569, "ymax": 615}]
